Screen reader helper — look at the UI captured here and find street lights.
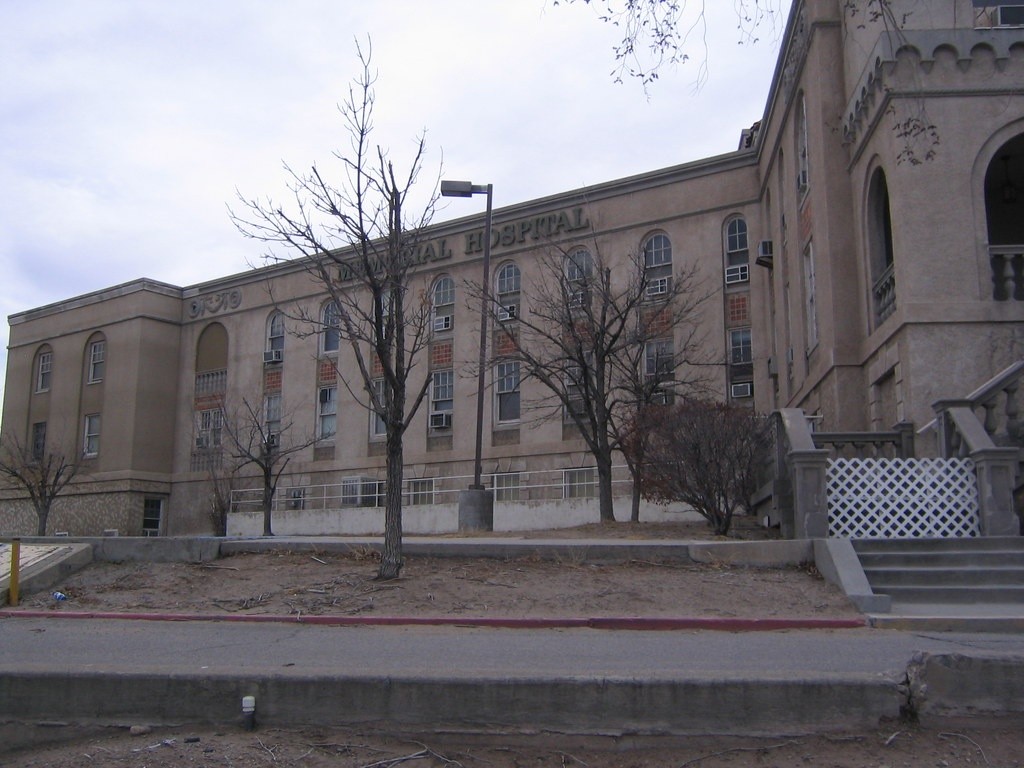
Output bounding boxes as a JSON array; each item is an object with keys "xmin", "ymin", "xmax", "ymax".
[{"xmin": 438, "ymin": 180, "xmax": 494, "ymax": 490}]
